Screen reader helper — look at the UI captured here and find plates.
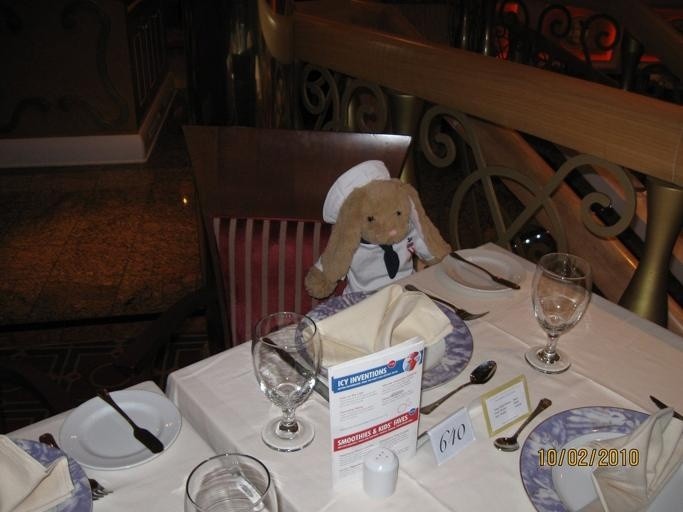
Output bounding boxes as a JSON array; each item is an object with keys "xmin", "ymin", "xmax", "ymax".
[
  {"xmin": 440, "ymin": 249, "xmax": 527, "ymax": 292},
  {"xmin": 8, "ymin": 438, "xmax": 93, "ymax": 512},
  {"xmin": 519, "ymin": 407, "xmax": 654, "ymax": 512},
  {"xmin": 295, "ymin": 291, "xmax": 474, "ymax": 391},
  {"xmin": 59, "ymin": 390, "xmax": 182, "ymax": 470}
]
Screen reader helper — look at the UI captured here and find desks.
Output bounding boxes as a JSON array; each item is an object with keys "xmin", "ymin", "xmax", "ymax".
[
  {"xmin": 165, "ymin": 236, "xmax": 683, "ymax": 512},
  {"xmin": 0, "ymin": 379, "xmax": 284, "ymax": 512}
]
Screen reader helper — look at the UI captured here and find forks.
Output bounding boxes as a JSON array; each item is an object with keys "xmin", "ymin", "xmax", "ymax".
[
  {"xmin": 404, "ymin": 283, "xmax": 490, "ymax": 321},
  {"xmin": 38, "ymin": 433, "xmax": 114, "ymax": 500}
]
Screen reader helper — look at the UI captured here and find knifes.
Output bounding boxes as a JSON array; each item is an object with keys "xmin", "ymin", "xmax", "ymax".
[
  {"xmin": 649, "ymin": 395, "xmax": 683, "ymax": 419},
  {"xmin": 263, "ymin": 338, "xmax": 329, "ymax": 401},
  {"xmin": 91, "ymin": 376, "xmax": 164, "ymax": 453},
  {"xmin": 449, "ymin": 251, "xmax": 520, "ymax": 290}
]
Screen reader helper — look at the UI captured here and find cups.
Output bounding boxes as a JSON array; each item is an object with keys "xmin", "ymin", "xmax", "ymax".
[{"xmin": 183, "ymin": 453, "xmax": 279, "ymax": 512}]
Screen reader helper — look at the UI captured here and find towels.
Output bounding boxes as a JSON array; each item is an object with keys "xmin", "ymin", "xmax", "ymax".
[
  {"xmin": 305, "ymin": 283, "xmax": 454, "ymax": 368},
  {"xmin": 591, "ymin": 406, "xmax": 683, "ymax": 512},
  {"xmin": 0, "ymin": 433, "xmax": 74, "ymax": 512},
  {"xmin": 347, "ymin": 238, "xmax": 412, "ymax": 293}
]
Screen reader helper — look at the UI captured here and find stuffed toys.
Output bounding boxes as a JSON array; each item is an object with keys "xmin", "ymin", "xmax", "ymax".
[{"xmin": 299, "ymin": 159, "xmax": 453, "ymax": 326}]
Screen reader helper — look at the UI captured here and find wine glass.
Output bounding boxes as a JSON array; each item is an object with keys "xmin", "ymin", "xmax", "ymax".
[
  {"xmin": 251, "ymin": 311, "xmax": 323, "ymax": 453},
  {"xmin": 525, "ymin": 253, "xmax": 592, "ymax": 374}
]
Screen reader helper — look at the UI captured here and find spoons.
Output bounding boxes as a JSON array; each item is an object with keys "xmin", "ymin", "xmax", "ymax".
[
  {"xmin": 416, "ymin": 360, "xmax": 497, "ymax": 413},
  {"xmin": 493, "ymin": 397, "xmax": 553, "ymax": 452}
]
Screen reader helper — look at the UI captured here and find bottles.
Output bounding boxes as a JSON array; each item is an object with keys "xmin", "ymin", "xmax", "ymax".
[{"xmin": 362, "ymin": 446, "xmax": 399, "ymax": 499}]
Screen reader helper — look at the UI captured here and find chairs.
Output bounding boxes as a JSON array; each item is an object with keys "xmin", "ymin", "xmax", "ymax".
[{"xmin": 181, "ymin": 126, "xmax": 412, "ymax": 356}]
